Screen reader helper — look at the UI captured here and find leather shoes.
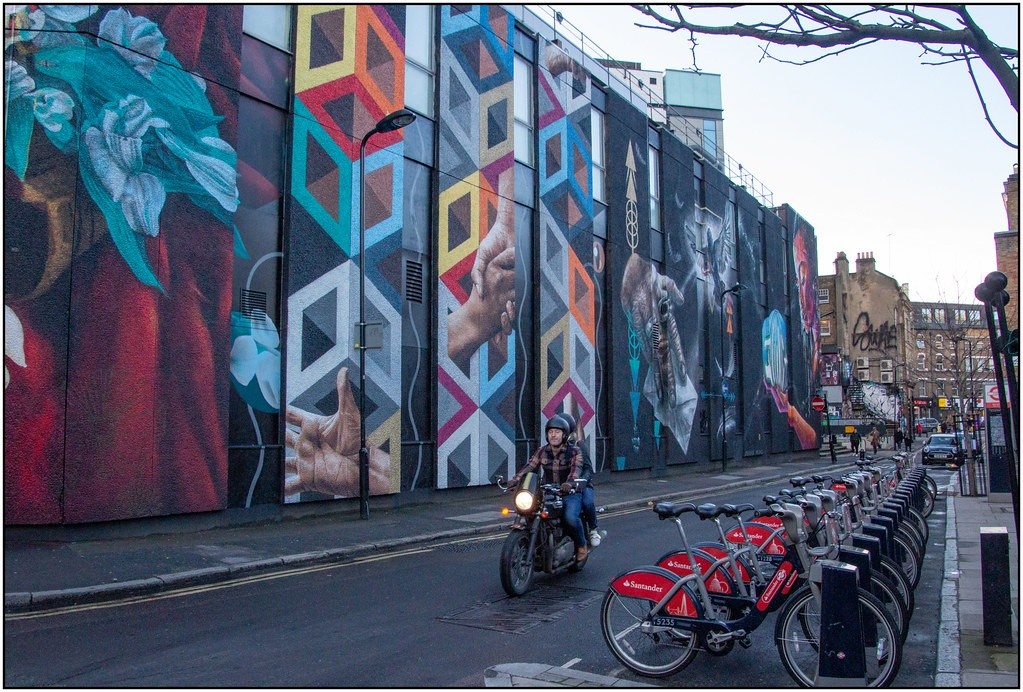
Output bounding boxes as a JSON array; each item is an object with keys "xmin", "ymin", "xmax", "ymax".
[{"xmin": 577, "ymin": 540, "xmax": 588, "ymax": 561}]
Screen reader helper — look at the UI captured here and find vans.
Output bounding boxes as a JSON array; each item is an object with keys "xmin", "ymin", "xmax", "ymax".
[{"xmin": 914, "ymin": 418, "xmax": 939, "ymax": 434}]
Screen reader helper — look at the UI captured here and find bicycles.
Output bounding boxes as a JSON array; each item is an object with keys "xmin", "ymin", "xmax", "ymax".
[{"xmin": 600, "ymin": 450, "xmax": 939, "ymax": 689}]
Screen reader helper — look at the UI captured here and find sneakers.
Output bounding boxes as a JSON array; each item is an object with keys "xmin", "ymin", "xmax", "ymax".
[{"xmin": 589, "ymin": 529, "xmax": 601, "ymax": 547}]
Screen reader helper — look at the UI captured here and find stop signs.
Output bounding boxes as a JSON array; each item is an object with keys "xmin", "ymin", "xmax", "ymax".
[{"xmin": 811, "ymin": 396, "xmax": 824, "ymax": 412}]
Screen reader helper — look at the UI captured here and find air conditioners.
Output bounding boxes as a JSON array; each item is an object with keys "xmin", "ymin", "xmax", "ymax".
[
  {"xmin": 880, "ymin": 359, "xmax": 894, "ymax": 372},
  {"xmin": 857, "ymin": 370, "xmax": 870, "ymax": 382},
  {"xmin": 857, "ymin": 356, "xmax": 870, "ymax": 369},
  {"xmin": 880, "ymin": 372, "xmax": 893, "ymax": 384}
]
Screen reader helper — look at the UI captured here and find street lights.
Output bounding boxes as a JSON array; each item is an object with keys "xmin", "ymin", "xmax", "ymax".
[
  {"xmin": 354, "ymin": 108, "xmax": 418, "ymax": 521},
  {"xmin": 719, "ymin": 283, "xmax": 749, "ymax": 471}
]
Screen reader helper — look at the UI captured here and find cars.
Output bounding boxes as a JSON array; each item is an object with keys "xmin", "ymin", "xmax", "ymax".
[{"xmin": 922, "ymin": 434, "xmax": 964, "ymax": 466}]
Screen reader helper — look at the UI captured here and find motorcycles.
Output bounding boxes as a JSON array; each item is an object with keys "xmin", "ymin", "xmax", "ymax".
[{"xmin": 494, "ymin": 473, "xmax": 608, "ymax": 596}]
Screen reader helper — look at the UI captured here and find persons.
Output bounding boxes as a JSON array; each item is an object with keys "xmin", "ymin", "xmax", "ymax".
[
  {"xmin": 541, "ymin": 413, "xmax": 601, "ymax": 547},
  {"xmin": 904, "ymin": 428, "xmax": 913, "ymax": 452},
  {"xmin": 507, "ymin": 416, "xmax": 587, "ymax": 561},
  {"xmin": 448, "ymin": 164, "xmax": 516, "ymax": 368},
  {"xmin": 939, "ymin": 420, "xmax": 952, "ymax": 433},
  {"xmin": 284, "ymin": 366, "xmax": 392, "ymax": 497},
  {"xmin": 893, "ymin": 428, "xmax": 904, "ymax": 450},
  {"xmin": 850, "ymin": 429, "xmax": 862, "ymax": 457},
  {"xmin": 916, "ymin": 419, "xmax": 923, "ymax": 438},
  {"xmin": 868, "ymin": 427, "xmax": 882, "ymax": 455}
]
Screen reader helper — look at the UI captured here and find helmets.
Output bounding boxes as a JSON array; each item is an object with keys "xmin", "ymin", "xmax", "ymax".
[
  {"xmin": 558, "ymin": 413, "xmax": 576, "ymax": 433},
  {"xmin": 545, "ymin": 415, "xmax": 569, "ymax": 443}
]
